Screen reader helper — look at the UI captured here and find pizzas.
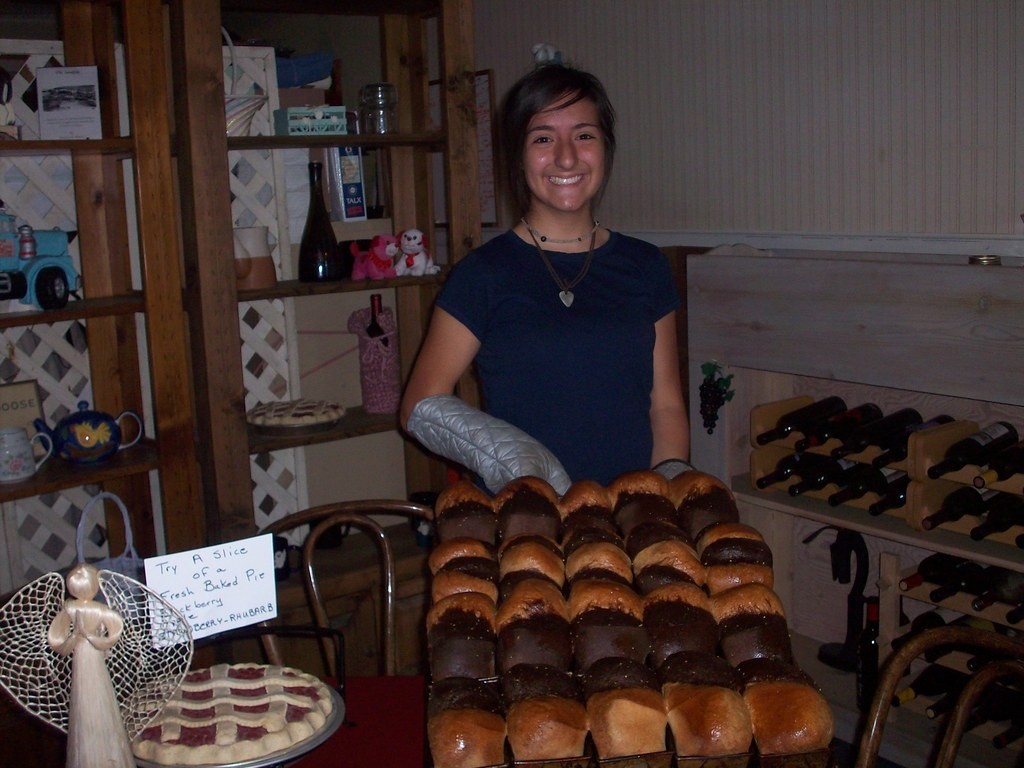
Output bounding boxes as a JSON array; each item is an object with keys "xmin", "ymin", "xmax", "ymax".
[
  {"xmin": 121, "ymin": 663, "xmax": 333, "ymax": 763},
  {"xmin": 246, "ymin": 398, "xmax": 346, "ymax": 426}
]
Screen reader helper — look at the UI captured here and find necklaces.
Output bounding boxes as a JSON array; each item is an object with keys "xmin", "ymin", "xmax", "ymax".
[{"xmin": 519, "ymin": 215, "xmax": 602, "ymax": 309}]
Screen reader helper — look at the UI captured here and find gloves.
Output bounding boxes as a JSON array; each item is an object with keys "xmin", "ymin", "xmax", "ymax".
[
  {"xmin": 406, "ymin": 394, "xmax": 573, "ymax": 496},
  {"xmin": 651, "ymin": 459, "xmax": 697, "ymax": 480}
]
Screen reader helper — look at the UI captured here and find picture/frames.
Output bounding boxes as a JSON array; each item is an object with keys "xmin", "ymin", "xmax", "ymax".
[{"xmin": 427, "ymin": 67, "xmax": 503, "ymax": 226}]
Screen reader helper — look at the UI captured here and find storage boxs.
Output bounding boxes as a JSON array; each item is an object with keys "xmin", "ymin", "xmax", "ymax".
[{"xmin": 329, "ymin": 218, "xmax": 393, "ymax": 278}]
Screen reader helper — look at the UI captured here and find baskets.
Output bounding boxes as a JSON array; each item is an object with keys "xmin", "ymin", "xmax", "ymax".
[
  {"xmin": 273, "ymin": 105, "xmax": 348, "ymax": 136},
  {"xmin": 76, "ymin": 492, "xmax": 147, "ymax": 621},
  {"xmin": 219, "ymin": 26, "xmax": 269, "ymax": 136}
]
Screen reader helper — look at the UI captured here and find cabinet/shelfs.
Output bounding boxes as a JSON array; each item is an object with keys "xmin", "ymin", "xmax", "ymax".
[
  {"xmin": 92, "ymin": 0, "xmax": 483, "ymax": 674},
  {"xmin": 688, "ymin": 246, "xmax": 1024, "ymax": 767},
  {"xmin": 0, "ymin": 0, "xmax": 218, "ymax": 669}
]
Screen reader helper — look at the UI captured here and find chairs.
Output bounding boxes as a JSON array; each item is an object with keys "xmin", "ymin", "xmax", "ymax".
[
  {"xmin": 254, "ymin": 498, "xmax": 436, "ymax": 675},
  {"xmin": 856, "ymin": 626, "xmax": 1024, "ymax": 768}
]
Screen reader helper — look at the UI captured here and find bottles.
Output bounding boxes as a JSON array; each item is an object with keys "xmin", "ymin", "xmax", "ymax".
[
  {"xmin": 856, "ymin": 596, "xmax": 880, "ymax": 716},
  {"xmin": 892, "ymin": 664, "xmax": 1024, "ymax": 751},
  {"xmin": 897, "ymin": 552, "xmax": 1024, "ymax": 625},
  {"xmin": 927, "ymin": 421, "xmax": 1019, "ymax": 481},
  {"xmin": 788, "ymin": 459, "xmax": 859, "ymax": 497},
  {"xmin": 298, "ymin": 160, "xmax": 343, "ymax": 283},
  {"xmin": 872, "ymin": 414, "xmax": 955, "ymax": 469},
  {"xmin": 756, "ymin": 396, "xmax": 847, "ymax": 444},
  {"xmin": 891, "ymin": 602, "xmax": 1024, "ymax": 686},
  {"xmin": 365, "ymin": 294, "xmax": 389, "ymax": 348},
  {"xmin": 970, "ymin": 494, "xmax": 1024, "ymax": 550},
  {"xmin": 973, "ymin": 438, "xmax": 1024, "ymax": 488},
  {"xmin": 869, "ymin": 472, "xmax": 910, "ymax": 517},
  {"xmin": 828, "ymin": 465, "xmax": 899, "ymax": 506},
  {"xmin": 831, "ymin": 407, "xmax": 923, "ymax": 461},
  {"xmin": 794, "ymin": 402, "xmax": 883, "ymax": 453},
  {"xmin": 922, "ymin": 485, "xmax": 1001, "ymax": 531},
  {"xmin": 756, "ymin": 452, "xmax": 811, "ymax": 489}
]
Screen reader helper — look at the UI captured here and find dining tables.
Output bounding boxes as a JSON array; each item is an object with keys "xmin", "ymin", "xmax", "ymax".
[{"xmin": 137, "ymin": 675, "xmax": 435, "ymax": 768}]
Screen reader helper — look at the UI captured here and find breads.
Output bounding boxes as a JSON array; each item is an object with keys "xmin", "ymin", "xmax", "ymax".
[{"xmin": 426, "ymin": 468, "xmax": 834, "ymax": 768}]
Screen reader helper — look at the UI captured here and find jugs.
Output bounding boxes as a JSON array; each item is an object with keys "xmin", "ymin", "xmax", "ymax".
[{"xmin": 232, "ymin": 226, "xmax": 277, "ymax": 291}]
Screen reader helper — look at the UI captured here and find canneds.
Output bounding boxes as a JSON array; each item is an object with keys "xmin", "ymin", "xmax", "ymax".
[{"xmin": 362, "ymin": 82, "xmax": 399, "ymax": 135}]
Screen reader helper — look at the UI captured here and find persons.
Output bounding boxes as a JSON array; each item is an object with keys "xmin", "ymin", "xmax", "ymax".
[{"xmin": 396, "ymin": 64, "xmax": 695, "ymax": 497}]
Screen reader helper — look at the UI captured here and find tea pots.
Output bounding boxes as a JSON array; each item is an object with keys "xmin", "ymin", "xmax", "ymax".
[
  {"xmin": 0, "ymin": 427, "xmax": 53, "ymax": 484},
  {"xmin": 33, "ymin": 400, "xmax": 142, "ymax": 464}
]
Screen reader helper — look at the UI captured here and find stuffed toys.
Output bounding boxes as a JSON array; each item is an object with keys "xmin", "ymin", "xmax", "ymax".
[
  {"xmin": 350, "ymin": 233, "xmax": 399, "ymax": 280},
  {"xmin": 394, "ymin": 227, "xmax": 441, "ymax": 277}
]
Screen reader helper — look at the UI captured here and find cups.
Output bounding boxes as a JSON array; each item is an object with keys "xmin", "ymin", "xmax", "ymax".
[
  {"xmin": 273, "ymin": 536, "xmax": 304, "ymax": 582},
  {"xmin": 309, "ymin": 516, "xmax": 351, "ymax": 548},
  {"xmin": 409, "ymin": 491, "xmax": 441, "ymax": 548}
]
